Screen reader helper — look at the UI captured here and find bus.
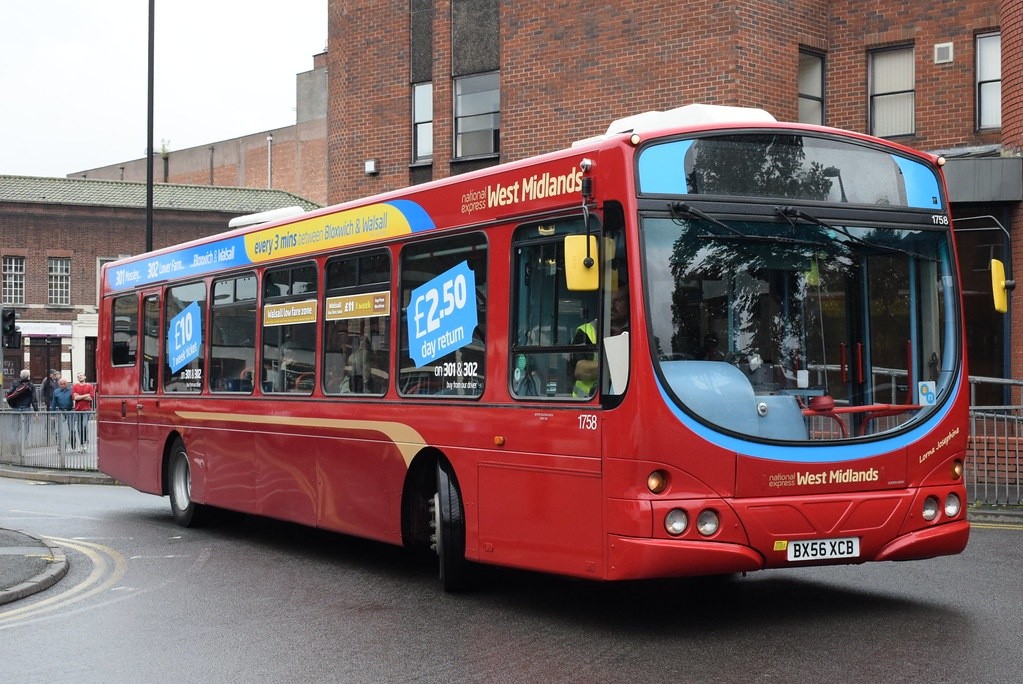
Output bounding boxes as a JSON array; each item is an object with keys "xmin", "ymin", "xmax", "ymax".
[{"xmin": 95, "ymin": 102, "xmax": 1017, "ymax": 597}]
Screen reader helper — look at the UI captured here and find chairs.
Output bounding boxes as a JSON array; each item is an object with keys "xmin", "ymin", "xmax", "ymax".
[
  {"xmin": 241, "ymin": 368, "xmax": 254, "ymax": 387},
  {"xmin": 407, "ymin": 382, "xmax": 441, "ymax": 397},
  {"xmin": 297, "ymin": 373, "xmax": 316, "ymax": 393}
]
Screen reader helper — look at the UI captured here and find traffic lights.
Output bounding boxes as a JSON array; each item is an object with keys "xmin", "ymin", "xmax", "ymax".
[
  {"xmin": 1, "ymin": 331, "xmax": 22, "ymax": 350},
  {"xmin": 0, "ymin": 308, "xmax": 15, "ymax": 337}
]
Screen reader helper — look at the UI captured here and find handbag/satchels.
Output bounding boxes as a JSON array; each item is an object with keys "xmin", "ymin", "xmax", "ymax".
[{"xmin": 5, "ymin": 379, "xmax": 32, "ymax": 408}]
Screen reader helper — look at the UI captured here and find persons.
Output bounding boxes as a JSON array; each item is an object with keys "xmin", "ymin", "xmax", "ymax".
[
  {"xmin": 5, "ymin": 370, "xmax": 39, "ymax": 455},
  {"xmin": 328, "ymin": 335, "xmax": 375, "ymax": 394},
  {"xmin": 278, "ymin": 338, "xmax": 297, "ymax": 392},
  {"xmin": 571, "ymin": 278, "xmax": 630, "ymax": 398},
  {"xmin": 40, "ymin": 369, "xmax": 94, "ymax": 454}
]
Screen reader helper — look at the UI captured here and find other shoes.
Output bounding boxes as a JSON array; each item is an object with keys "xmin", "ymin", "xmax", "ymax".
[{"xmin": 82, "ymin": 444, "xmax": 87, "ymax": 450}]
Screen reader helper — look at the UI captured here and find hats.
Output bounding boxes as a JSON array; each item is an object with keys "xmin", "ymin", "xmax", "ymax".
[{"xmin": 49, "ymin": 368, "xmax": 57, "ymax": 374}]
